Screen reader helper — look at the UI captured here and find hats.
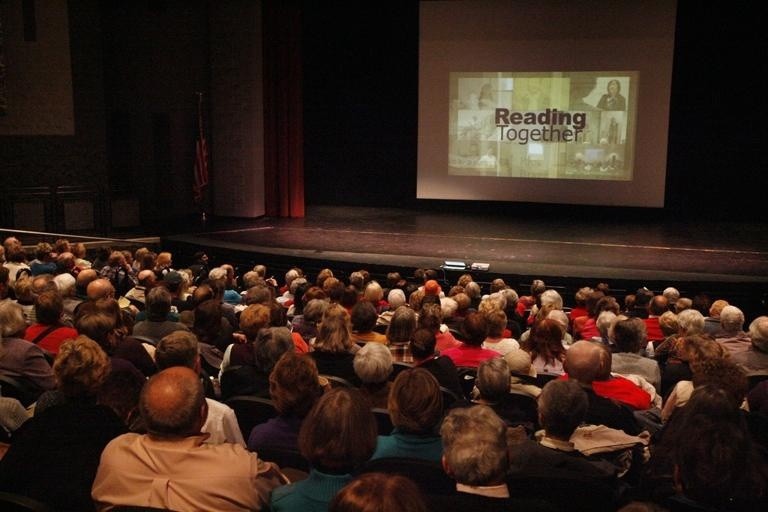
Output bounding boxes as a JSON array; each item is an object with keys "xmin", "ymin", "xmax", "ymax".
[
  {"xmin": 421, "ymin": 278, "xmax": 444, "ymax": 298},
  {"xmin": 208, "ymin": 266, "xmax": 228, "ymax": 282}
]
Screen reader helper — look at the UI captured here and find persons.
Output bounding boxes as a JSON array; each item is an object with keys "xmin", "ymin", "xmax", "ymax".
[
  {"xmin": 478, "ymin": 83, "xmax": 496, "ymax": 110},
  {"xmin": 373, "ymin": 269, "xmax": 767, "ymax": 512},
  {"xmin": 1, "ymin": 237, "xmax": 426, "ymax": 511},
  {"xmin": 596, "ymin": 79, "xmax": 626, "ymax": 111},
  {"xmin": 562, "ymin": 72, "xmax": 603, "ymax": 111}
]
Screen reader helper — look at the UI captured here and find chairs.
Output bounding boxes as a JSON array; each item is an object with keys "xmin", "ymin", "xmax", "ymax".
[{"xmin": 1, "ymin": 264, "xmax": 765, "ymax": 512}]
